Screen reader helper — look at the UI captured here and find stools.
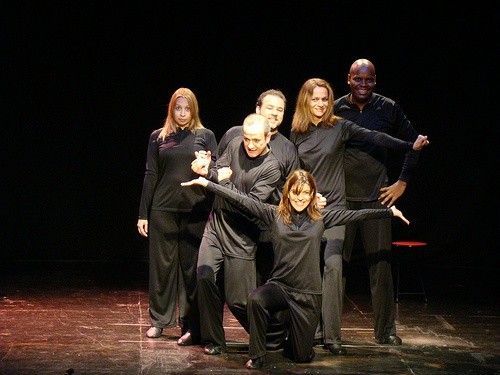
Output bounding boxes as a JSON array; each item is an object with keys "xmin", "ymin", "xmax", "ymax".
[{"xmin": 392, "ymin": 241, "xmax": 429, "ymax": 303}]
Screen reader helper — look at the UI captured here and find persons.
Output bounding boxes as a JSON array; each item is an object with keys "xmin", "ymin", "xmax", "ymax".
[
  {"xmin": 191, "ymin": 114, "xmax": 281, "ymax": 356},
  {"xmin": 314, "ymin": 59, "xmax": 423, "ymax": 346},
  {"xmin": 289, "ymin": 78, "xmax": 429, "ymax": 355},
  {"xmin": 137, "ymin": 88, "xmax": 217, "ymax": 338},
  {"xmin": 195, "ymin": 88, "xmax": 300, "ymax": 350},
  {"xmin": 180, "ymin": 169, "xmax": 410, "ymax": 368}
]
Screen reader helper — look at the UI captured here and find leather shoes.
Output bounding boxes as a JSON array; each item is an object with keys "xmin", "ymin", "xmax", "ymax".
[
  {"xmin": 177, "ymin": 331, "xmax": 194, "ymax": 345},
  {"xmin": 324, "ymin": 342, "xmax": 347, "ymax": 356},
  {"xmin": 380, "ymin": 335, "xmax": 402, "ymax": 346},
  {"xmin": 146, "ymin": 327, "xmax": 162, "ymax": 338},
  {"xmin": 246, "ymin": 357, "xmax": 264, "ymax": 369},
  {"xmin": 204, "ymin": 345, "xmax": 218, "ymax": 354},
  {"xmin": 313, "ymin": 338, "xmax": 324, "ymax": 346}
]
